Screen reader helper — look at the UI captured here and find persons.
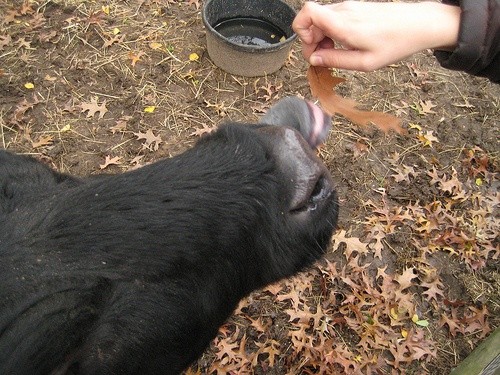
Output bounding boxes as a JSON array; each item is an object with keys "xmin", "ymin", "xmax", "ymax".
[{"xmin": 293, "ymin": 0, "xmax": 500, "ymax": 85}]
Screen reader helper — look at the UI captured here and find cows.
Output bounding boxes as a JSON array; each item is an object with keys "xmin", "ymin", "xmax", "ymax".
[{"xmin": 0, "ymin": 96, "xmax": 341, "ymax": 375}]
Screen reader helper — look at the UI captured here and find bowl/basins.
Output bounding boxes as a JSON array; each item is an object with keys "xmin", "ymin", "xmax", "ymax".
[{"xmin": 202, "ymin": 0, "xmax": 299, "ymax": 78}]
